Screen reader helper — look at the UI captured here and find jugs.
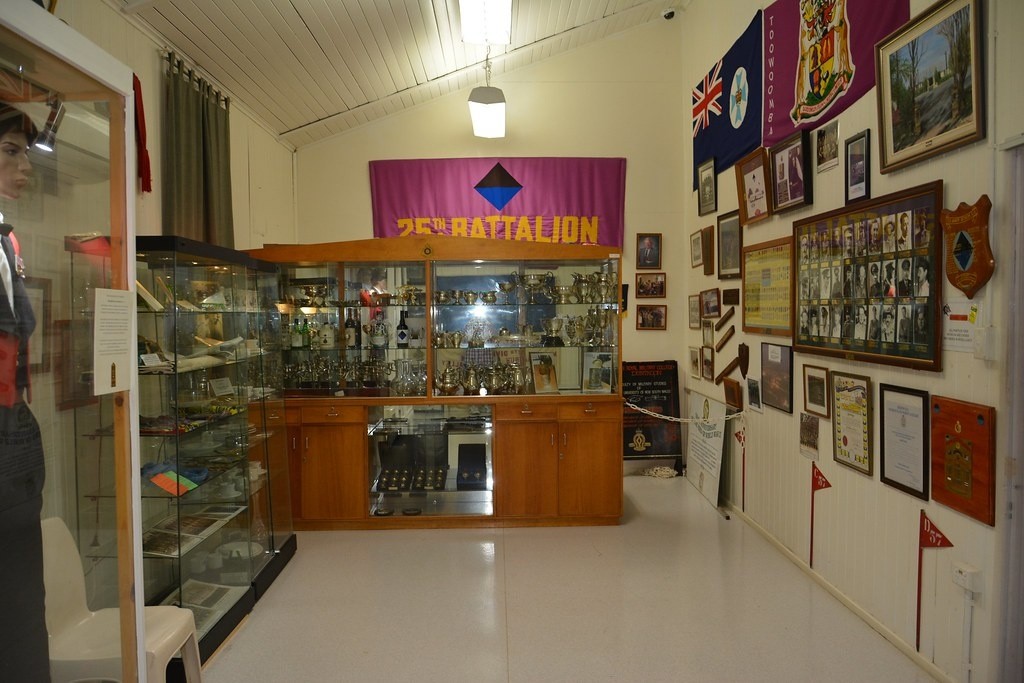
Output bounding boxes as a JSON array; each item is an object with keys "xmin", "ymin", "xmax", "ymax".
[{"xmin": 479, "ymin": 291, "xmax": 498, "ymax": 305}]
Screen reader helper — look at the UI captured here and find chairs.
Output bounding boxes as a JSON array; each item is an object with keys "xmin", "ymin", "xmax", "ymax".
[{"xmin": 41, "ymin": 515, "xmax": 209, "ymax": 682}]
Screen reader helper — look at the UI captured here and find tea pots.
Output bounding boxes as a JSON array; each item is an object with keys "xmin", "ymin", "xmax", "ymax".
[{"xmin": 434, "ymin": 360, "xmax": 524, "ymax": 394}]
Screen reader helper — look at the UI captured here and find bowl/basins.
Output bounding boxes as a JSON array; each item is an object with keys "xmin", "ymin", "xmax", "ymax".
[{"xmin": 169, "ymin": 391, "xmax": 217, "ymax": 419}]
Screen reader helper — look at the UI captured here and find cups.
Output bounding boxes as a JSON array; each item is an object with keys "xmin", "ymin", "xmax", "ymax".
[
  {"xmin": 437, "ymin": 290, "xmax": 478, "ymax": 305},
  {"xmin": 233, "ymin": 386, "xmax": 270, "ymax": 397},
  {"xmin": 590, "ymin": 368, "xmax": 601, "ymax": 386},
  {"xmin": 190, "ymin": 551, "xmax": 223, "ymax": 573},
  {"xmin": 244, "ymin": 339, "xmax": 260, "ymax": 349},
  {"xmin": 245, "ymin": 467, "xmax": 258, "ymax": 482},
  {"xmin": 249, "ymin": 461, "xmax": 261, "ymax": 473},
  {"xmin": 220, "ymin": 482, "xmax": 235, "ymax": 496},
  {"xmin": 540, "ymin": 318, "xmax": 563, "ymax": 337},
  {"xmin": 225, "ymin": 434, "xmax": 246, "ymax": 448}
]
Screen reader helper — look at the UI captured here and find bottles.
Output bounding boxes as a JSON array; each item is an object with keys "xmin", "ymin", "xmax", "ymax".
[
  {"xmin": 343, "ymin": 308, "xmax": 356, "ymax": 350},
  {"xmin": 404, "ymin": 311, "xmax": 408, "ymax": 327},
  {"xmin": 354, "ymin": 308, "xmax": 361, "ymax": 349},
  {"xmin": 291, "ymin": 318, "xmax": 310, "ymax": 349},
  {"xmin": 539, "ymin": 361, "xmax": 551, "ymax": 384},
  {"xmin": 397, "ymin": 310, "xmax": 408, "ymax": 348},
  {"xmin": 184, "ymin": 370, "xmax": 209, "ymax": 401},
  {"xmin": 392, "ymin": 359, "xmax": 427, "ymax": 395},
  {"xmin": 319, "ymin": 322, "xmax": 335, "ymax": 349}
]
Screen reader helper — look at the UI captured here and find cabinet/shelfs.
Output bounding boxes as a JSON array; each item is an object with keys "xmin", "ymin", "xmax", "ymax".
[
  {"xmin": 255, "ymin": 236, "xmax": 629, "ymax": 526},
  {"xmin": 68, "ymin": 239, "xmax": 290, "ymax": 665}
]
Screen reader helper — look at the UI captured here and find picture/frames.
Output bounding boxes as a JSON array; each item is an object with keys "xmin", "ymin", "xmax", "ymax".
[
  {"xmin": 801, "ymin": 364, "xmax": 829, "ymax": 417},
  {"xmin": 878, "ymin": 1, "xmax": 987, "ymax": 174},
  {"xmin": 878, "ymin": 379, "xmax": 930, "ymax": 501},
  {"xmin": 794, "ymin": 182, "xmax": 948, "ymax": 372},
  {"xmin": 635, "ymin": 232, "xmax": 667, "ymax": 330},
  {"xmin": 741, "ymin": 234, "xmax": 798, "ymax": 334},
  {"xmin": 830, "ymin": 369, "xmax": 874, "ymax": 477},
  {"xmin": 757, "ymin": 340, "xmax": 792, "ymax": 415},
  {"xmin": 746, "ymin": 375, "xmax": 764, "ymax": 414},
  {"xmin": 695, "ymin": 161, "xmax": 719, "ymax": 215},
  {"xmin": 769, "ymin": 127, "xmax": 815, "ymax": 214},
  {"xmin": 735, "ymin": 147, "xmax": 772, "ymax": 225},
  {"xmin": 717, "ymin": 209, "xmax": 743, "ymax": 278},
  {"xmin": 24, "ymin": 275, "xmax": 52, "ymax": 375},
  {"xmin": 841, "ymin": 128, "xmax": 873, "ymax": 203},
  {"xmin": 686, "ymin": 286, "xmax": 720, "ymax": 380},
  {"xmin": 688, "ymin": 226, "xmax": 714, "ymax": 274},
  {"xmin": 52, "ymin": 318, "xmax": 98, "ymax": 409}
]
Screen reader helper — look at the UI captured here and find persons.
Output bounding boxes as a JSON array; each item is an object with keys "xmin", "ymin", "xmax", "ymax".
[
  {"xmin": 643, "ymin": 310, "xmax": 663, "ymax": 326},
  {"xmin": 801, "ymin": 305, "xmax": 926, "ymax": 342},
  {"xmin": 640, "ymin": 236, "xmax": 659, "ymax": 266},
  {"xmin": 645, "ymin": 277, "xmax": 661, "ymax": 294},
  {"xmin": 801, "ymin": 259, "xmax": 931, "ymax": 298},
  {"xmin": 788, "ymin": 147, "xmax": 804, "ymax": 196},
  {"xmin": 800, "ymin": 207, "xmax": 932, "ymax": 264},
  {"xmin": 0, "ymin": 99, "xmax": 54, "ymax": 683}
]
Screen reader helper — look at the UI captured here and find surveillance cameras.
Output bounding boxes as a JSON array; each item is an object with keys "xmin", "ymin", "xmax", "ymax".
[{"xmin": 661, "ymin": 8, "xmax": 675, "ymax": 20}]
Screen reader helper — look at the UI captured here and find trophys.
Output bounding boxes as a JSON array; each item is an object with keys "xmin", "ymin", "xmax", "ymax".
[
  {"xmin": 434, "ymin": 329, "xmax": 485, "ymax": 348},
  {"xmin": 539, "ymin": 305, "xmax": 615, "ymax": 347},
  {"xmin": 450, "ymin": 289, "xmax": 465, "ymax": 305},
  {"xmin": 436, "ymin": 360, "xmax": 524, "ymax": 395},
  {"xmin": 435, "ymin": 290, "xmax": 451, "ymax": 305},
  {"xmin": 479, "ymin": 290, "xmax": 497, "ymax": 304},
  {"xmin": 291, "ymin": 355, "xmax": 395, "ymax": 387},
  {"xmin": 544, "ymin": 281, "xmax": 578, "ymax": 304},
  {"xmin": 464, "ymin": 290, "xmax": 478, "ymax": 305},
  {"xmin": 571, "ymin": 272, "xmax": 617, "ymax": 304},
  {"xmin": 498, "ymin": 281, "xmax": 516, "ymax": 305},
  {"xmin": 509, "ymin": 270, "xmax": 553, "ymax": 305}
]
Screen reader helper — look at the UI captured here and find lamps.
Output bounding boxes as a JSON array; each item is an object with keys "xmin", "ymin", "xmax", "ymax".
[
  {"xmin": 35, "ymin": 98, "xmax": 66, "ymax": 154},
  {"xmin": 467, "ymin": 46, "xmax": 507, "ymax": 142}
]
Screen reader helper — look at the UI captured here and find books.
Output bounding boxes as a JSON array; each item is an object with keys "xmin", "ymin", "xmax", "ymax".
[{"xmin": 136, "ymin": 281, "xmax": 165, "ymax": 312}]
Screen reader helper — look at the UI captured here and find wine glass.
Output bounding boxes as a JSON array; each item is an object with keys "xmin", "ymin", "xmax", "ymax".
[
  {"xmin": 555, "ymin": 286, "xmax": 573, "ymax": 305},
  {"xmin": 498, "ymin": 281, "xmax": 516, "ymax": 304},
  {"xmin": 512, "ymin": 271, "xmax": 553, "ymax": 305}
]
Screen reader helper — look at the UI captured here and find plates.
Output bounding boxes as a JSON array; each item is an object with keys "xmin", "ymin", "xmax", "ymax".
[
  {"xmin": 183, "ymin": 442, "xmax": 222, "ymax": 456},
  {"xmin": 214, "ymin": 490, "xmax": 242, "ymax": 498},
  {"xmin": 257, "ymin": 469, "xmax": 267, "ymax": 475},
  {"xmin": 215, "ymin": 542, "xmax": 263, "ymax": 559}
]
